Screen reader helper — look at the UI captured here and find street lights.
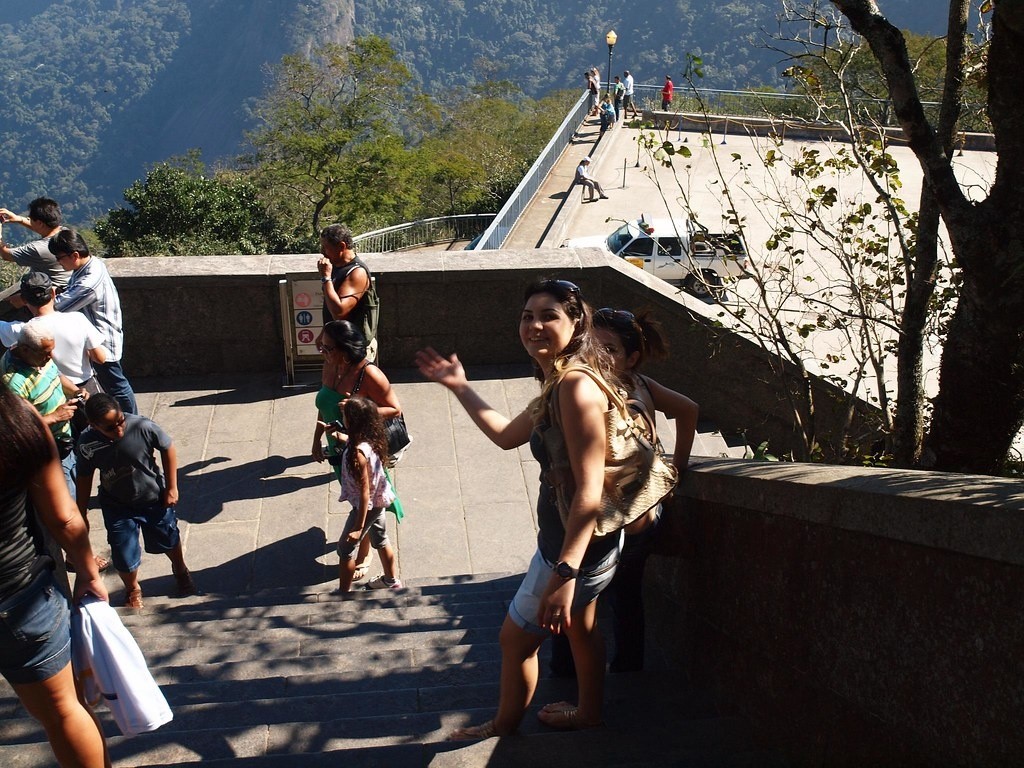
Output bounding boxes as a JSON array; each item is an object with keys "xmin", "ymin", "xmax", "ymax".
[{"xmin": 606, "ymin": 29, "xmax": 618, "ymax": 94}]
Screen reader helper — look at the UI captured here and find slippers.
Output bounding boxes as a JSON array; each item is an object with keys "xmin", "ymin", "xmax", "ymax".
[{"xmin": 352, "ymin": 558, "xmax": 372, "ymax": 580}]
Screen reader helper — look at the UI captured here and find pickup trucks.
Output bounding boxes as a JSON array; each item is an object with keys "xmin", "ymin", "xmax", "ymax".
[{"xmin": 556, "ymin": 212, "xmax": 752, "ymax": 299}]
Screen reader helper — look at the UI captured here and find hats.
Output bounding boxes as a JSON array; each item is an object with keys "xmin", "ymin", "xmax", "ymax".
[
  {"xmin": 21, "ymin": 272, "xmax": 52, "ymax": 299},
  {"xmin": 583, "ymin": 157, "xmax": 592, "ymax": 162}
]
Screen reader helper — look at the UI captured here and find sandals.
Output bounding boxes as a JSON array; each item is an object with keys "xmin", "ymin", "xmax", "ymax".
[
  {"xmin": 66, "ymin": 554, "xmax": 109, "ymax": 572},
  {"xmin": 538, "ymin": 700, "xmax": 596, "ymax": 730},
  {"xmin": 447, "ymin": 719, "xmax": 500, "ymax": 742}
]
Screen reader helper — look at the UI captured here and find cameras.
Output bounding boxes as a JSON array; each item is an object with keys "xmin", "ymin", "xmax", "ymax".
[
  {"xmin": 0, "ymin": 214, "xmax": 5, "ymax": 223},
  {"xmin": 327, "ymin": 420, "xmax": 343, "ymax": 431},
  {"xmin": 68, "ymin": 393, "xmax": 86, "ymax": 409}
]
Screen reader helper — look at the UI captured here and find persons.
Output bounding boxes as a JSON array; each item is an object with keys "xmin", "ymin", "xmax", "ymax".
[
  {"xmin": 584, "ymin": 67, "xmax": 600, "ymax": 116},
  {"xmin": 576, "ymin": 157, "xmax": 609, "ymax": 202},
  {"xmin": 414, "ymin": 279, "xmax": 626, "ymax": 740},
  {"xmin": 0, "ymin": 368, "xmax": 113, "ymax": 768},
  {"xmin": 312, "ymin": 320, "xmax": 401, "ymax": 580},
  {"xmin": 329, "ymin": 398, "xmax": 402, "ymax": 593},
  {"xmin": 1, "ymin": 197, "xmax": 137, "ymax": 572},
  {"xmin": 76, "ymin": 393, "xmax": 206, "ymax": 607},
  {"xmin": 318, "ymin": 223, "xmax": 380, "ymax": 368},
  {"xmin": 660, "ymin": 75, "xmax": 673, "ymax": 112},
  {"xmin": 613, "ymin": 76, "xmax": 626, "ymax": 122},
  {"xmin": 549, "ymin": 308, "xmax": 698, "ymax": 683},
  {"xmin": 622, "ymin": 70, "xmax": 638, "ymax": 119},
  {"xmin": 597, "ymin": 93, "xmax": 617, "ymax": 139}
]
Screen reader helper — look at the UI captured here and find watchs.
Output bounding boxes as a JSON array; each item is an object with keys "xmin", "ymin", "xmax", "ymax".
[
  {"xmin": 553, "ymin": 560, "xmax": 579, "ymax": 580},
  {"xmin": 321, "ymin": 277, "xmax": 332, "ymax": 283}
]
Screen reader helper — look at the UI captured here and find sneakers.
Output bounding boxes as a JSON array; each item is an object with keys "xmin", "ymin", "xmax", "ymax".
[
  {"xmin": 328, "ymin": 588, "xmax": 342, "ymax": 593},
  {"xmin": 368, "ymin": 576, "xmax": 401, "ymax": 590}
]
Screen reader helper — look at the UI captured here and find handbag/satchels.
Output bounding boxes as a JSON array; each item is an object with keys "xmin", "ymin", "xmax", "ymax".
[
  {"xmin": 351, "ymin": 362, "xmax": 411, "ymax": 456},
  {"xmin": 543, "ymin": 367, "xmax": 680, "ymax": 542}
]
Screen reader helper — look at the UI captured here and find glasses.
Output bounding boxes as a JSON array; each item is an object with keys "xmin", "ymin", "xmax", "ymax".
[
  {"xmin": 93, "ymin": 414, "xmax": 128, "ymax": 433},
  {"xmin": 540, "ymin": 278, "xmax": 581, "ymax": 314},
  {"xmin": 319, "ymin": 341, "xmax": 339, "ymax": 354},
  {"xmin": 595, "ymin": 308, "xmax": 634, "ymax": 322},
  {"xmin": 56, "ymin": 254, "xmax": 69, "ymax": 261}
]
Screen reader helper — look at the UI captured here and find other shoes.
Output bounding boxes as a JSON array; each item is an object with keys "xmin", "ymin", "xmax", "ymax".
[
  {"xmin": 590, "ymin": 198, "xmax": 598, "ymax": 201},
  {"xmin": 600, "ymin": 196, "xmax": 609, "ymax": 199},
  {"xmin": 590, "ymin": 113, "xmax": 598, "ymax": 116},
  {"xmin": 604, "ymin": 662, "xmax": 613, "ymax": 673},
  {"xmin": 624, "ymin": 117, "xmax": 627, "ymax": 119},
  {"xmin": 171, "ymin": 562, "xmax": 196, "ymax": 594},
  {"xmin": 632, "ymin": 114, "xmax": 638, "ymax": 118},
  {"xmin": 125, "ymin": 588, "xmax": 144, "ymax": 608}
]
